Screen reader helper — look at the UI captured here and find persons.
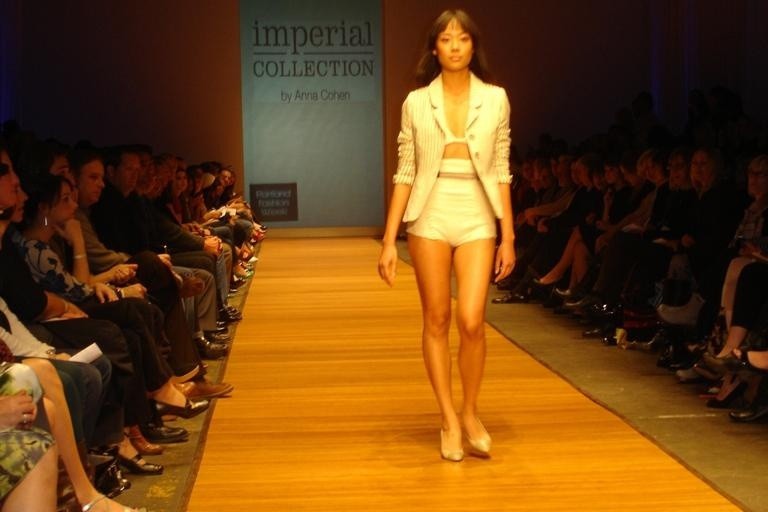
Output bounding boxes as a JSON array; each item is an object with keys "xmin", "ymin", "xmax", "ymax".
[
  {"xmin": 0, "ymin": 120, "xmax": 268, "ymax": 512},
  {"xmin": 377, "ymin": 9, "xmax": 517, "ymax": 463},
  {"xmin": 490, "ymin": 84, "xmax": 767, "ymax": 424}
]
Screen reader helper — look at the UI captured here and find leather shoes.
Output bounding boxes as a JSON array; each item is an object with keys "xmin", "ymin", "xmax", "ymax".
[
  {"xmin": 532, "ymin": 278, "xmax": 638, "ymax": 346},
  {"xmin": 196, "ymin": 304, "xmax": 242, "ymax": 359},
  {"xmin": 493, "ymin": 276, "xmax": 529, "ymax": 303},
  {"xmin": 440, "ymin": 426, "xmax": 464, "ymax": 461},
  {"xmin": 464, "ymin": 415, "xmax": 492, "ymax": 456},
  {"xmin": 228, "ymin": 223, "xmax": 267, "ymax": 298},
  {"xmin": 641, "ymin": 291, "xmax": 768, "ymax": 422},
  {"xmin": 118, "ymin": 379, "xmax": 234, "ymax": 476}
]
